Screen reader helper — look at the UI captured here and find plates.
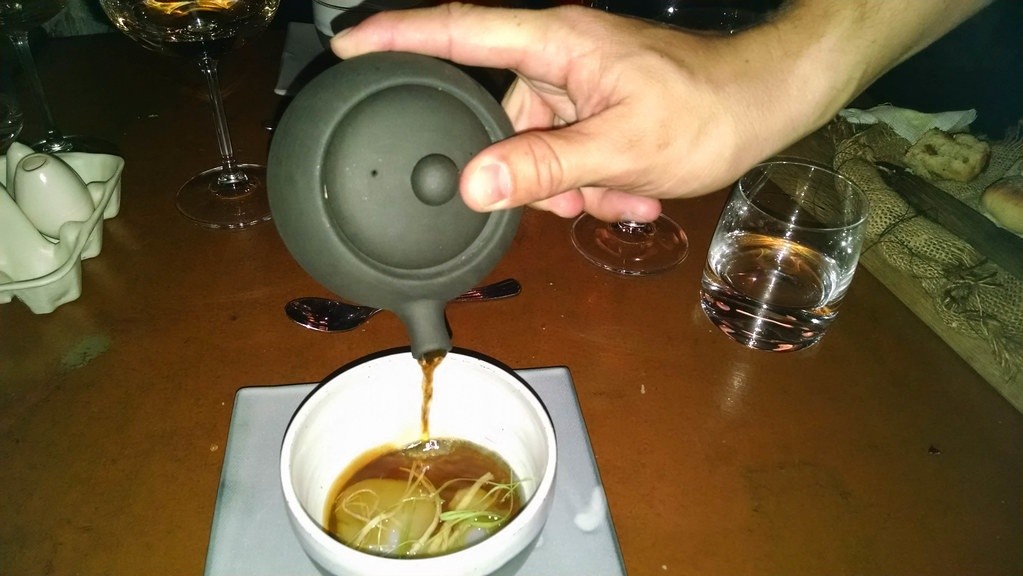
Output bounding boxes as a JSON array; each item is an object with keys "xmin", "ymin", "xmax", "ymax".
[
  {"xmin": 200, "ymin": 365, "xmax": 629, "ymax": 576},
  {"xmin": 272, "ymin": 20, "xmax": 323, "ymax": 97}
]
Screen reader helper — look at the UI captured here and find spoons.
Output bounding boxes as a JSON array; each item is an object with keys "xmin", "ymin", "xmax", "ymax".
[{"xmin": 283, "ymin": 276, "xmax": 523, "ymax": 335}]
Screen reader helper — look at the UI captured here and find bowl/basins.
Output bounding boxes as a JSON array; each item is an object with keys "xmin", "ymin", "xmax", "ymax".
[{"xmin": 312, "ymin": 0, "xmax": 425, "ymax": 55}]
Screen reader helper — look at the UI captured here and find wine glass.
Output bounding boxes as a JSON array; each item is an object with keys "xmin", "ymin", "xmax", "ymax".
[
  {"xmin": 0, "ymin": 0, "xmax": 121, "ymax": 157},
  {"xmin": 570, "ymin": 1, "xmax": 753, "ymax": 277},
  {"xmin": 100, "ymin": 0, "xmax": 273, "ymax": 229}
]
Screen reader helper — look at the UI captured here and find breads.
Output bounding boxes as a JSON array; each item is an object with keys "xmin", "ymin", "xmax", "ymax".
[
  {"xmin": 905, "ymin": 127, "xmax": 991, "ymax": 184},
  {"xmin": 983, "ymin": 174, "xmax": 1023, "ymax": 235}
]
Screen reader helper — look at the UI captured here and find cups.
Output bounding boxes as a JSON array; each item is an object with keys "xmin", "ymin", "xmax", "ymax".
[
  {"xmin": 698, "ymin": 161, "xmax": 870, "ymax": 354},
  {"xmin": 277, "ymin": 351, "xmax": 559, "ymax": 575}
]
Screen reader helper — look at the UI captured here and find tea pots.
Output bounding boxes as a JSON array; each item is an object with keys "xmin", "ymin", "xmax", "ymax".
[{"xmin": 264, "ymin": 51, "xmax": 528, "ymax": 362}]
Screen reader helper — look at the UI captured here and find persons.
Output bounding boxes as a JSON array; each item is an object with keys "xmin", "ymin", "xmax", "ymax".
[{"xmin": 331, "ymin": 0, "xmax": 1023, "ymax": 223}]
